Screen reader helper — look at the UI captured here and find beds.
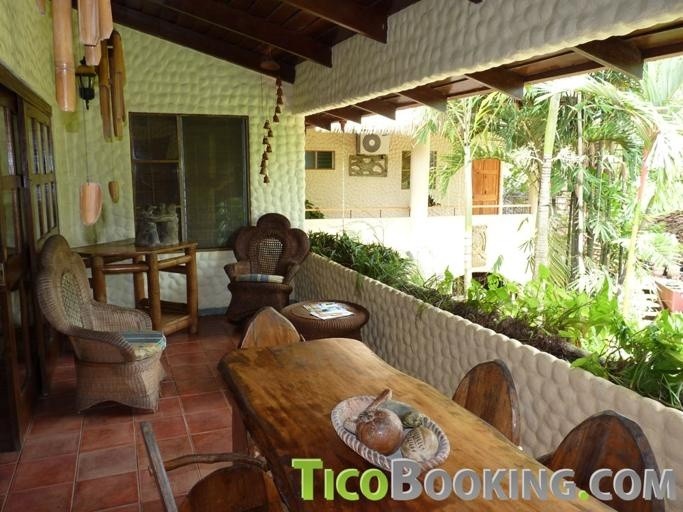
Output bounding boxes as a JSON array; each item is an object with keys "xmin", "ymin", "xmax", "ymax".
[{"xmin": 283, "ymin": 299, "xmax": 369, "ymax": 342}]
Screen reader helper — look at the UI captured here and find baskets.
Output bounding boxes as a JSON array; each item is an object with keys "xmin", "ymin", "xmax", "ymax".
[{"xmin": 333, "ymin": 394, "xmax": 451, "ymax": 475}]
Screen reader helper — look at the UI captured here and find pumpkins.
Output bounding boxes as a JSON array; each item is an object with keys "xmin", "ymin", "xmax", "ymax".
[{"xmin": 346, "ymin": 387, "xmax": 437, "ymax": 464}]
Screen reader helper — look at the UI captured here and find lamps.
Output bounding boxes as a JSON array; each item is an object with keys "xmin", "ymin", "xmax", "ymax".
[{"xmin": 75, "ymin": 56, "xmax": 96, "ymax": 111}]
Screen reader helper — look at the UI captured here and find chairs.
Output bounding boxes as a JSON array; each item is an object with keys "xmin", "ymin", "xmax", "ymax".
[
  {"xmin": 221, "ymin": 213, "xmax": 310, "ymax": 322},
  {"xmin": 139, "ymin": 306, "xmax": 666, "ymax": 510},
  {"xmin": 36, "ymin": 235, "xmax": 168, "ymax": 412}
]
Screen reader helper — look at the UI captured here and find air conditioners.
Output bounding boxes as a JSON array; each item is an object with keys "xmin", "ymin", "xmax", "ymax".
[{"xmin": 355, "ymin": 133, "xmax": 390, "ymax": 156}]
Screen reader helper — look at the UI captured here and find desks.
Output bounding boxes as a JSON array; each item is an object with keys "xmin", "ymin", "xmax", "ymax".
[{"xmin": 72, "ymin": 237, "xmax": 198, "ymax": 336}]
